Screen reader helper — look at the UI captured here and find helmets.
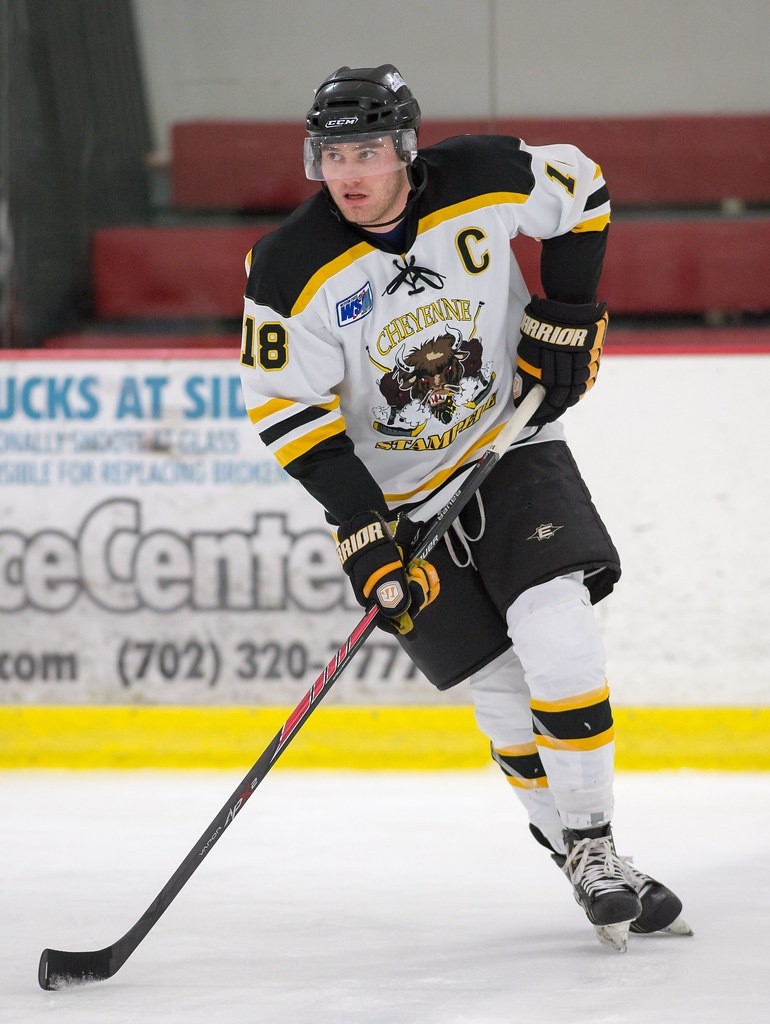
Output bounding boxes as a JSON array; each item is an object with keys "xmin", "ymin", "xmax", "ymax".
[{"xmin": 304, "ymin": 63, "xmax": 422, "ymax": 166}]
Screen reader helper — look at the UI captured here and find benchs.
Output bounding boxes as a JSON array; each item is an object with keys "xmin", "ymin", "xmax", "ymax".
[{"xmin": 95, "ymin": 115, "xmax": 770, "ymax": 325}]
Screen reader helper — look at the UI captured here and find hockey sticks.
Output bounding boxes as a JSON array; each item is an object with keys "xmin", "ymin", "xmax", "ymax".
[{"xmin": 36, "ymin": 380, "xmax": 548, "ymax": 994}]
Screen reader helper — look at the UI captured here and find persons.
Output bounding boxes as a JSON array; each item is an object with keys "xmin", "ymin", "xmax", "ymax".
[{"xmin": 237, "ymin": 64, "xmax": 693, "ymax": 954}]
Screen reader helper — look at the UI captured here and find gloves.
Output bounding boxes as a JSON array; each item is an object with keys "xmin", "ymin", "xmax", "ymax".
[
  {"xmin": 323, "ymin": 513, "xmax": 439, "ymax": 639},
  {"xmin": 514, "ymin": 294, "xmax": 609, "ymax": 425}
]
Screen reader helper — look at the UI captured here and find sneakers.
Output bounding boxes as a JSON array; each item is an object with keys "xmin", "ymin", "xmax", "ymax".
[
  {"xmin": 528, "ymin": 822, "xmax": 694, "ymax": 938},
  {"xmin": 561, "ymin": 821, "xmax": 641, "ymax": 954}
]
